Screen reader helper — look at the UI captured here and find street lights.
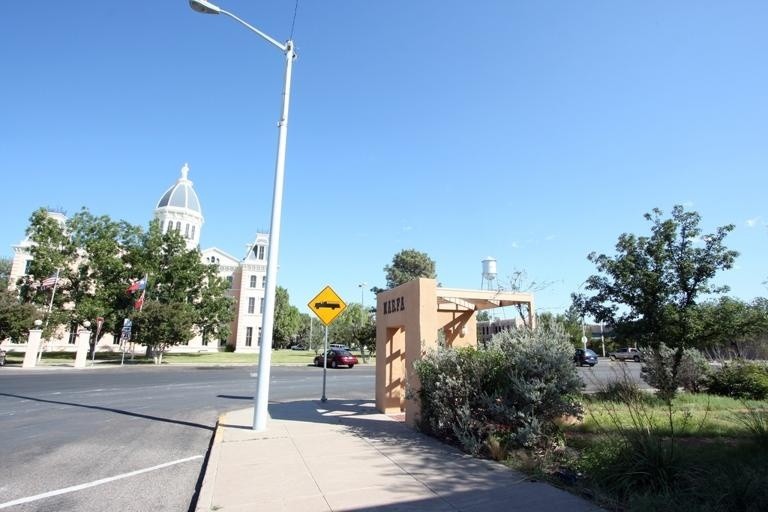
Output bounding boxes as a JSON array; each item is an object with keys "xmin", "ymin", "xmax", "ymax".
[
  {"xmin": 359, "ymin": 284, "xmax": 364, "ymax": 326},
  {"xmin": 189, "ymin": 1, "xmax": 297, "ymax": 431}
]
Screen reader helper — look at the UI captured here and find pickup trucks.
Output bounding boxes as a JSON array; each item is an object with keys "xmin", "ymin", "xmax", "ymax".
[{"xmin": 608, "ymin": 347, "xmax": 642, "ymax": 361}]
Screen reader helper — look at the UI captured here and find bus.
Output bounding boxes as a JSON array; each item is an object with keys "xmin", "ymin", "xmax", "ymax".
[{"xmin": 329, "ymin": 344, "xmax": 346, "ymax": 350}]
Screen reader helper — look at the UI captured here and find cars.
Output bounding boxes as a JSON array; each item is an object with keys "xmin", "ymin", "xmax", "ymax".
[
  {"xmin": 573, "ymin": 348, "xmax": 598, "ymax": 366},
  {"xmin": 313, "ymin": 349, "xmax": 358, "ymax": 368},
  {"xmin": 291, "ymin": 344, "xmax": 306, "ymax": 350}
]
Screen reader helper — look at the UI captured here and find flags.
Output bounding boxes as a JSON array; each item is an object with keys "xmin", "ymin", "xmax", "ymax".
[
  {"xmin": 134, "ymin": 290, "xmax": 144, "ymax": 312},
  {"xmin": 42, "ymin": 270, "xmax": 57, "ymax": 286},
  {"xmin": 126, "ymin": 276, "xmax": 146, "ymax": 294}
]
